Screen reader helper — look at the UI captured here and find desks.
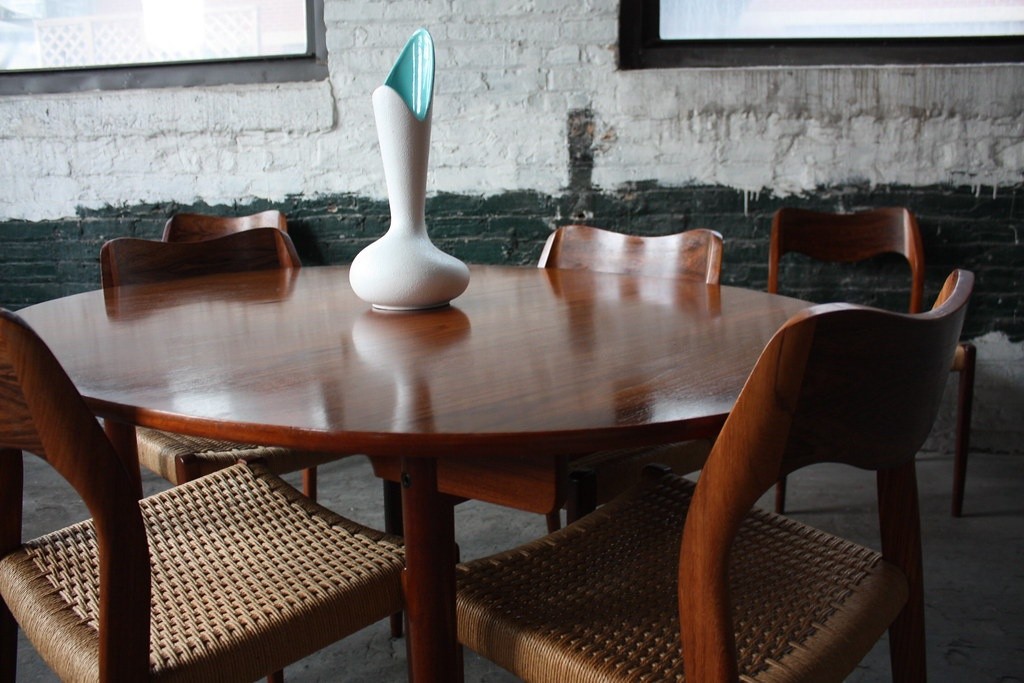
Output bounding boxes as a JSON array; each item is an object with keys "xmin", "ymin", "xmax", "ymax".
[{"xmin": 0, "ymin": 266, "xmax": 816, "ymax": 683}]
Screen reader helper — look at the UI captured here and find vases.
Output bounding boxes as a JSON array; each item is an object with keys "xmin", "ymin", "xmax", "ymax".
[{"xmin": 349, "ymin": 28, "xmax": 468, "ymax": 311}]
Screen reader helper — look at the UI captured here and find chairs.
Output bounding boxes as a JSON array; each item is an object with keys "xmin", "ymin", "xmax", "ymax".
[
  {"xmin": 456, "ymin": 270, "xmax": 977, "ymax": 683},
  {"xmin": 0, "ymin": 308, "xmax": 459, "ymax": 683},
  {"xmin": 368, "ymin": 226, "xmax": 725, "ymax": 643},
  {"xmin": 766, "ymin": 208, "xmax": 976, "ymax": 516},
  {"xmin": 161, "ymin": 209, "xmax": 287, "ymax": 242},
  {"xmin": 98, "ymin": 229, "xmax": 360, "ymax": 503}
]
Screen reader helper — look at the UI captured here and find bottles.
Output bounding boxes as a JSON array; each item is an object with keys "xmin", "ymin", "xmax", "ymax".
[{"xmin": 348, "ymin": 28, "xmax": 471, "ymax": 313}]
